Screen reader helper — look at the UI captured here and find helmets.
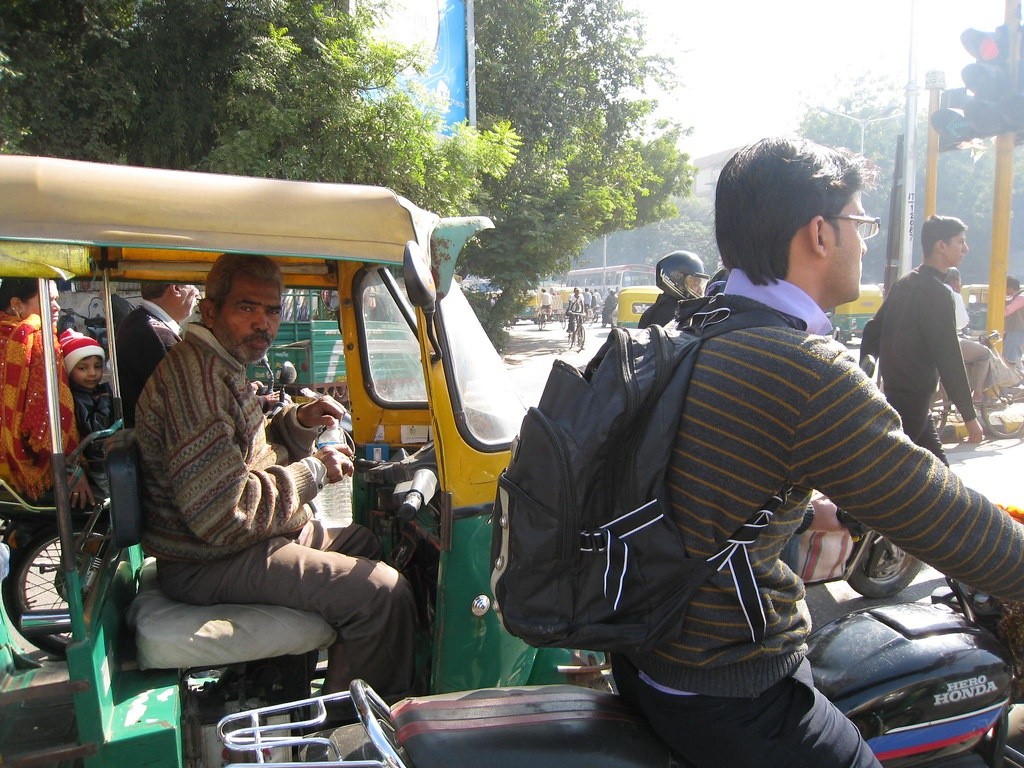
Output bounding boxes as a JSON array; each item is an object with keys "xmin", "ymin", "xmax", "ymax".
[{"xmin": 656, "ymin": 251, "xmax": 710, "ymax": 298}]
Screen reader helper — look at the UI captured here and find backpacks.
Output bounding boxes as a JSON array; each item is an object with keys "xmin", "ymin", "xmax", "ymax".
[{"xmin": 490, "ymin": 294, "xmax": 808, "ymax": 674}]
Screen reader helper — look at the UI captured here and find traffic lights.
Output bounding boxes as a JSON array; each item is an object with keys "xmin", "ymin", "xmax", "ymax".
[{"xmin": 933, "ymin": 25, "xmax": 1016, "ymax": 151}]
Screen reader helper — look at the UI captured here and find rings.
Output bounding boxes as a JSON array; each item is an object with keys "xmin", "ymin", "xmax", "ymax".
[{"xmin": 74, "ymin": 492, "xmax": 79, "ymax": 494}]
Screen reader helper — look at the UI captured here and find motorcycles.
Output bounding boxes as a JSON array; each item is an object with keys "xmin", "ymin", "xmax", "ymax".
[
  {"xmin": 617, "ymin": 286, "xmax": 664, "ymax": 328},
  {"xmin": 831, "ymin": 283, "xmax": 990, "ymax": 345},
  {"xmin": 0, "ymin": 156, "xmax": 607, "ymax": 768},
  {"xmin": 3, "ymin": 354, "xmax": 299, "ymax": 659},
  {"xmin": 489, "ymin": 288, "xmax": 577, "ymax": 325},
  {"xmin": 799, "ymin": 354, "xmax": 924, "ymax": 598},
  {"xmin": 214, "ymin": 503, "xmax": 1024, "ymax": 768}
]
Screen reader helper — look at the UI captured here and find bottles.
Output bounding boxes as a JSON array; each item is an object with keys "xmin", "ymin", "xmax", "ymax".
[{"xmin": 317, "ymin": 418, "xmax": 353, "ymax": 528}]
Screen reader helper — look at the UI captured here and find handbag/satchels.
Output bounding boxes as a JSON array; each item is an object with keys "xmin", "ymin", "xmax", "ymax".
[
  {"xmin": 798, "ymin": 529, "xmax": 854, "ymax": 583},
  {"xmin": 990, "ymin": 348, "xmax": 1023, "ymax": 388},
  {"xmin": 565, "ymin": 308, "xmax": 572, "ymax": 316}
]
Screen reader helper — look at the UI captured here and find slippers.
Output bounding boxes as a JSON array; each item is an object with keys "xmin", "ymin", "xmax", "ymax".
[{"xmin": 976, "ymin": 399, "xmax": 998, "ymax": 406}]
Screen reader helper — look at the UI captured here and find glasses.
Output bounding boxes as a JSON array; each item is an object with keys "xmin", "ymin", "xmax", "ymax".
[{"xmin": 823, "ymin": 214, "xmax": 881, "ymax": 239}]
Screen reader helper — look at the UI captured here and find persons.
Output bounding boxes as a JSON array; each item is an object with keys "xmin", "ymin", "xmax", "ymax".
[
  {"xmin": 611, "ymin": 136, "xmax": 1024, "ymax": 768},
  {"xmin": 1003, "ymin": 276, "xmax": 1024, "ymax": 401},
  {"xmin": 944, "ymin": 267, "xmax": 994, "ymax": 406},
  {"xmin": 638, "ymin": 251, "xmax": 709, "ymax": 329},
  {"xmin": 537, "ymin": 288, "xmax": 558, "ymax": 321},
  {"xmin": 488, "ymin": 293, "xmax": 497, "ymax": 307},
  {"xmin": 0, "ymin": 277, "xmax": 96, "ymax": 508},
  {"xmin": 137, "ymin": 253, "xmax": 419, "ymax": 732},
  {"xmin": 60, "ymin": 326, "xmax": 113, "ymax": 464},
  {"xmin": 566, "ymin": 287, "xmax": 618, "ymax": 338},
  {"xmin": 859, "ymin": 214, "xmax": 983, "ymax": 468},
  {"xmin": 119, "ymin": 280, "xmax": 292, "ymax": 427}
]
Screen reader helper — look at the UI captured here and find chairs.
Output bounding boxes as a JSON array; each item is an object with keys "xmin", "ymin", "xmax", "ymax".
[{"xmin": 103, "ymin": 426, "xmax": 336, "ymax": 768}]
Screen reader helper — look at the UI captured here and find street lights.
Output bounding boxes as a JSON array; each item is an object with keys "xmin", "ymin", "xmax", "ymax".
[{"xmin": 812, "ymin": 104, "xmax": 905, "ymax": 156}]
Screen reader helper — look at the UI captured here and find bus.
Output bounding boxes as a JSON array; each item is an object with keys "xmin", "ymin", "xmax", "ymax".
[{"xmin": 566, "ymin": 264, "xmax": 656, "ymax": 299}]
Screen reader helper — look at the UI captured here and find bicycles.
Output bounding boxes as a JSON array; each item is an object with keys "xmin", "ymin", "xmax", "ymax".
[
  {"xmin": 928, "ymin": 330, "xmax": 1024, "ymax": 440},
  {"xmin": 533, "ymin": 304, "xmax": 613, "ymax": 349}
]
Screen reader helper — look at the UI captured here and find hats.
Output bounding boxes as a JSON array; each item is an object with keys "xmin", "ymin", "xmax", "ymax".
[
  {"xmin": 60, "ymin": 328, "xmax": 106, "ymax": 377},
  {"xmin": 574, "ymin": 287, "xmax": 579, "ymax": 291}
]
